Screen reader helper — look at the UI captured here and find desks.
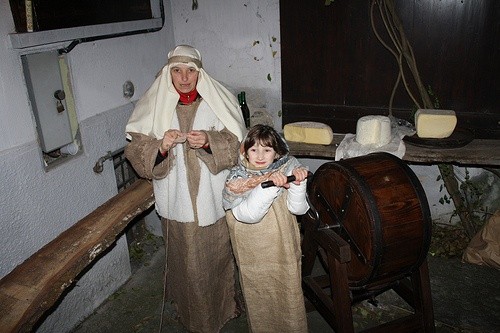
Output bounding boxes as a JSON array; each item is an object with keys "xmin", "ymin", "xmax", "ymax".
[
  {"xmin": 0, "ymin": 178, "xmax": 155, "ymax": 333},
  {"xmin": 280, "ymin": 132, "xmax": 500, "ymax": 243}
]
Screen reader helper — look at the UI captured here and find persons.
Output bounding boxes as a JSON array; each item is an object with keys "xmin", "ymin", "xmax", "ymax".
[
  {"xmin": 222, "ymin": 124, "xmax": 310, "ymax": 333},
  {"xmin": 124, "ymin": 45, "xmax": 248, "ymax": 333}
]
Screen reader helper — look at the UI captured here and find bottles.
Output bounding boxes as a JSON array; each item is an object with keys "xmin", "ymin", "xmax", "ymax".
[
  {"xmin": 238, "ymin": 94, "xmax": 247, "ymax": 127},
  {"xmin": 241, "ymin": 92, "xmax": 251, "ymax": 127}
]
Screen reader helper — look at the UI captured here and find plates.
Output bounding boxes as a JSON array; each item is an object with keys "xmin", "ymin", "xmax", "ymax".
[{"xmin": 404, "ymin": 126, "xmax": 477, "ymax": 148}]
